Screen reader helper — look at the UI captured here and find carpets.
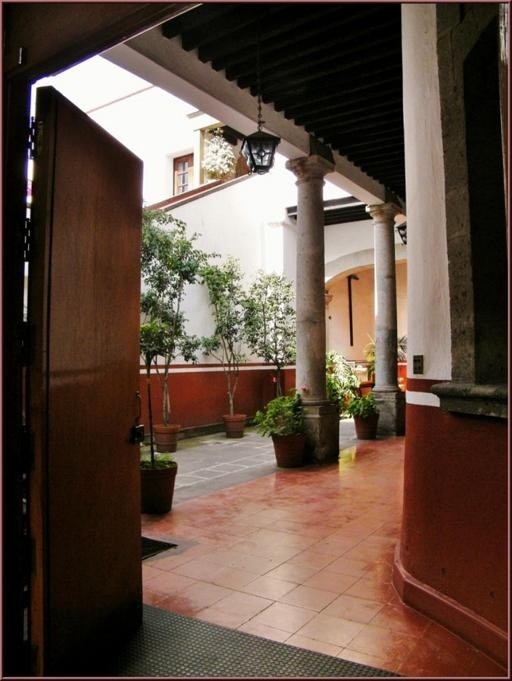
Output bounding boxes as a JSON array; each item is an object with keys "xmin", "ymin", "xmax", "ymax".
[{"xmin": 142, "ymin": 535, "xmax": 179, "ymax": 561}]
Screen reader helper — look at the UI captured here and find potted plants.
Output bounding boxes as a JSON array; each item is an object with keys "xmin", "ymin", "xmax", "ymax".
[
  {"xmin": 350, "ymin": 392, "xmax": 381, "ymax": 440},
  {"xmin": 253, "ymin": 394, "xmax": 314, "ymax": 468},
  {"xmin": 140, "ymin": 319, "xmax": 178, "ymax": 517}
]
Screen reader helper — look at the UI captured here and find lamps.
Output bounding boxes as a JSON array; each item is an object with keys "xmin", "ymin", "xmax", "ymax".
[
  {"xmin": 240, "ymin": 47, "xmax": 279, "ymax": 173},
  {"xmin": 397, "ymin": 221, "xmax": 407, "ymax": 244}
]
